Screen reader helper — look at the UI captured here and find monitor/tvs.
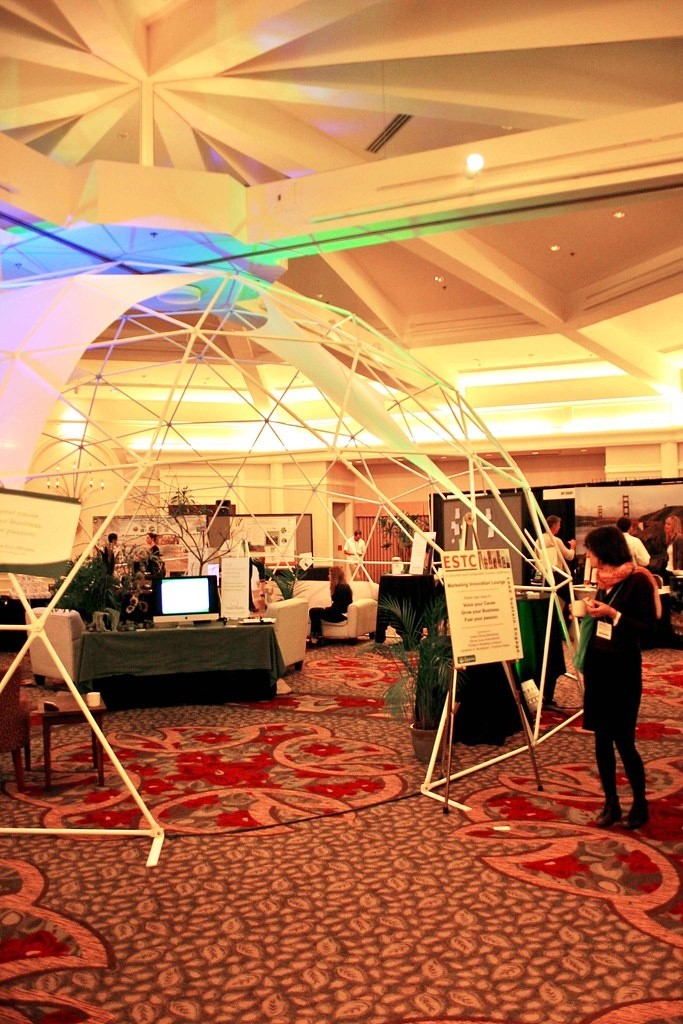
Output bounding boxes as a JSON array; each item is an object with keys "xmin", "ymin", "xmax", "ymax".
[{"xmin": 153, "ymin": 575, "xmax": 219, "ymax": 628}]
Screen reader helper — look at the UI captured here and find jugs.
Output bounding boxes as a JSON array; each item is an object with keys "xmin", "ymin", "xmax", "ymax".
[{"xmin": 391, "ymin": 557, "xmax": 402, "ymax": 575}]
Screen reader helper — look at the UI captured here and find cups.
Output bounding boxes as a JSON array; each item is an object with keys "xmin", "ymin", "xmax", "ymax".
[
  {"xmin": 571, "ymin": 600, "xmax": 586, "ymax": 617},
  {"xmin": 87, "ymin": 692, "xmax": 100, "ymax": 707}
]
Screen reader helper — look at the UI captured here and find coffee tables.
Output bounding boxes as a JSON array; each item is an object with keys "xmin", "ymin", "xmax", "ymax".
[{"xmin": 37, "ymin": 692, "xmax": 107, "ymax": 788}]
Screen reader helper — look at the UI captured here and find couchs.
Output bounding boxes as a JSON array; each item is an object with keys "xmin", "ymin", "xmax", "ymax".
[
  {"xmin": 264, "ymin": 597, "xmax": 309, "ymax": 670},
  {"xmin": 25, "ymin": 607, "xmax": 86, "ymax": 686},
  {"xmin": 261, "ymin": 582, "xmax": 382, "ymax": 645},
  {"xmin": 0, "ymin": 667, "xmax": 31, "ymax": 792}
]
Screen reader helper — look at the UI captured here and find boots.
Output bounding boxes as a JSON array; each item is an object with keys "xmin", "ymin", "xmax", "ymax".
[
  {"xmin": 596, "ymin": 795, "xmax": 622, "ymax": 827},
  {"xmin": 621, "ymin": 798, "xmax": 649, "ymax": 830}
]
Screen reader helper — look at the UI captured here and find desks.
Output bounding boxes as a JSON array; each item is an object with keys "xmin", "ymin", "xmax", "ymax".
[{"xmin": 80, "ymin": 619, "xmax": 287, "ymax": 701}]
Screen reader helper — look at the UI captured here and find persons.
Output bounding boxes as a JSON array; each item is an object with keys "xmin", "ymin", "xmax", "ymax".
[
  {"xmin": 343, "ymin": 530, "xmax": 365, "ymax": 580},
  {"xmin": 306, "ymin": 566, "xmax": 352, "ymax": 647},
  {"xmin": 534, "ymin": 515, "xmax": 577, "ymax": 603},
  {"xmin": 616, "ymin": 517, "xmax": 650, "ymax": 569},
  {"xmin": 145, "ymin": 533, "xmax": 160, "ymax": 555},
  {"xmin": 665, "ymin": 516, "xmax": 683, "ymax": 576},
  {"xmin": 103, "ymin": 533, "xmax": 117, "ymax": 574},
  {"xmin": 569, "ymin": 526, "xmax": 658, "ymax": 832}
]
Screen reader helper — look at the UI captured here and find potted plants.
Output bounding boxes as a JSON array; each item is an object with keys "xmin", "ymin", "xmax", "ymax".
[{"xmin": 354, "ymin": 587, "xmax": 470, "ymax": 761}]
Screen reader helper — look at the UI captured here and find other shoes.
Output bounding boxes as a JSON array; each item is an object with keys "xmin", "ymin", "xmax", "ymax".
[{"xmin": 307, "ymin": 638, "xmax": 323, "ymax": 648}]
